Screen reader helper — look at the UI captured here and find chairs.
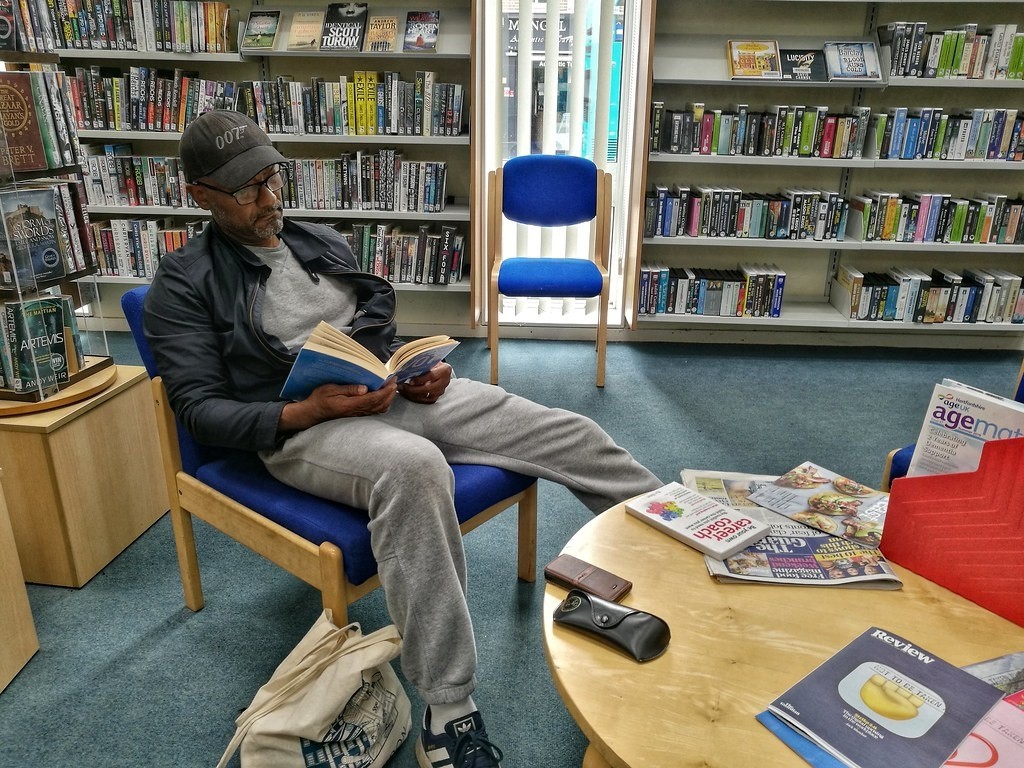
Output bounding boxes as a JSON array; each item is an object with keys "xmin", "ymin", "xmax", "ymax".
[
  {"xmin": 880, "ymin": 356, "xmax": 1024, "ymax": 492},
  {"xmin": 484, "ymin": 151, "xmax": 617, "ymax": 395},
  {"xmin": 118, "ymin": 282, "xmax": 544, "ymax": 633}
]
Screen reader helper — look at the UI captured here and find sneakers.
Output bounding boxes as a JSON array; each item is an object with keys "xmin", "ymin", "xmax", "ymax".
[{"xmin": 415, "ymin": 703, "xmax": 504, "ymax": 768}]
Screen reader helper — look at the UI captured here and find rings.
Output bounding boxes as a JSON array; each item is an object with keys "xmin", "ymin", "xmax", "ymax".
[{"xmin": 425, "ymin": 392, "xmax": 430, "ymax": 398}]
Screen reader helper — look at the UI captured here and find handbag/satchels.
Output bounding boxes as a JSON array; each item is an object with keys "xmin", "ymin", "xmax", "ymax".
[{"xmin": 215, "ymin": 607, "xmax": 413, "ymax": 768}]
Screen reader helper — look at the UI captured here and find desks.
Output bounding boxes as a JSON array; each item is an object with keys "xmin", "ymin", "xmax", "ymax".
[{"xmin": 539, "ymin": 480, "xmax": 1024, "ymax": 768}]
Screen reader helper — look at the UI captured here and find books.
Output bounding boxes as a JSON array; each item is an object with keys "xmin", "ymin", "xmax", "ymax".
[
  {"xmin": 835, "ymin": 262, "xmax": 1024, "ymax": 326},
  {"xmin": 638, "ymin": 261, "xmax": 786, "ymax": 318},
  {"xmin": 624, "ymin": 480, "xmax": 770, "ymax": 561},
  {"xmin": 642, "ymin": 182, "xmax": 1024, "ymax": 246},
  {"xmin": 0, "ymin": 0, "xmax": 471, "ymax": 401},
  {"xmin": 278, "ymin": 319, "xmax": 461, "ymax": 399},
  {"xmin": 878, "ymin": 21, "xmax": 1024, "ymax": 81},
  {"xmin": 649, "ymin": 101, "xmax": 1024, "ymax": 162},
  {"xmin": 905, "ymin": 376, "xmax": 1023, "ymax": 478}
]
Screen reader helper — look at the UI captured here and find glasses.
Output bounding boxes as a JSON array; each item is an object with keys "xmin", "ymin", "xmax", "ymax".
[{"xmin": 191, "ymin": 163, "xmax": 290, "ymax": 206}]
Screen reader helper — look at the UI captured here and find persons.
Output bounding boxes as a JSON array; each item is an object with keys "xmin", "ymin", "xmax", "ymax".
[{"xmin": 140, "ymin": 109, "xmax": 663, "ymax": 767}]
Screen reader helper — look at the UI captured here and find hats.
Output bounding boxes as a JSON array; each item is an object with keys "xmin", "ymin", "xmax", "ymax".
[{"xmin": 179, "ymin": 108, "xmax": 290, "ymax": 189}]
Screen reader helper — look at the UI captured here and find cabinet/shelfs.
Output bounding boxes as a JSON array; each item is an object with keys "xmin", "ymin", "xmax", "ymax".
[
  {"xmin": 37, "ymin": 0, "xmax": 481, "ymax": 336},
  {"xmin": 629, "ymin": 0, "xmax": 1024, "ymax": 341},
  {"xmin": 38, "ymin": 164, "xmax": 48, "ymax": 171},
  {"xmin": 0, "ymin": 0, "xmax": 128, "ymax": 421}
]
